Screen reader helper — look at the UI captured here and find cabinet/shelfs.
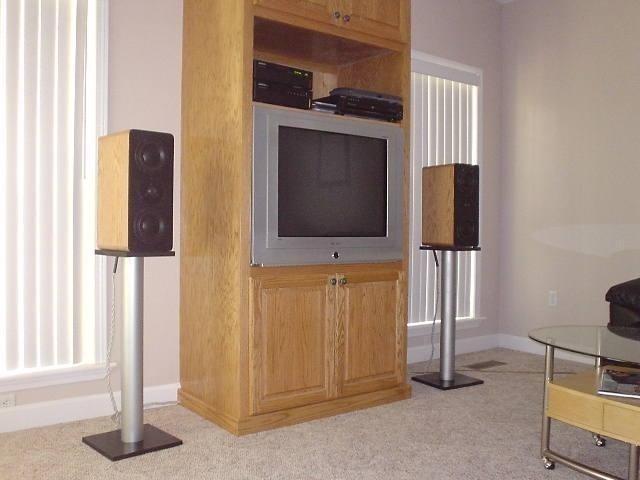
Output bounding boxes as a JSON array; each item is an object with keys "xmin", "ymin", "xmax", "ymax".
[{"xmin": 179, "ymin": 1, "xmax": 417, "ymax": 435}]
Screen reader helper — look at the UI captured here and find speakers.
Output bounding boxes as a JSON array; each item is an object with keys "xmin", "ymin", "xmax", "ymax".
[
  {"xmin": 422, "ymin": 164, "xmax": 479, "ymax": 247},
  {"xmin": 97, "ymin": 129, "xmax": 175, "ymax": 252}
]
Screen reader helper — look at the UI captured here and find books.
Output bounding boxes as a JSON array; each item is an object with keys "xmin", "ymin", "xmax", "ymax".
[{"xmin": 598, "ymin": 368, "xmax": 640, "ymax": 399}]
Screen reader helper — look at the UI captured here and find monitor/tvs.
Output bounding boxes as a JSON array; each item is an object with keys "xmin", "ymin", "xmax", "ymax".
[{"xmin": 252, "ymin": 101, "xmax": 404, "ymax": 268}]
